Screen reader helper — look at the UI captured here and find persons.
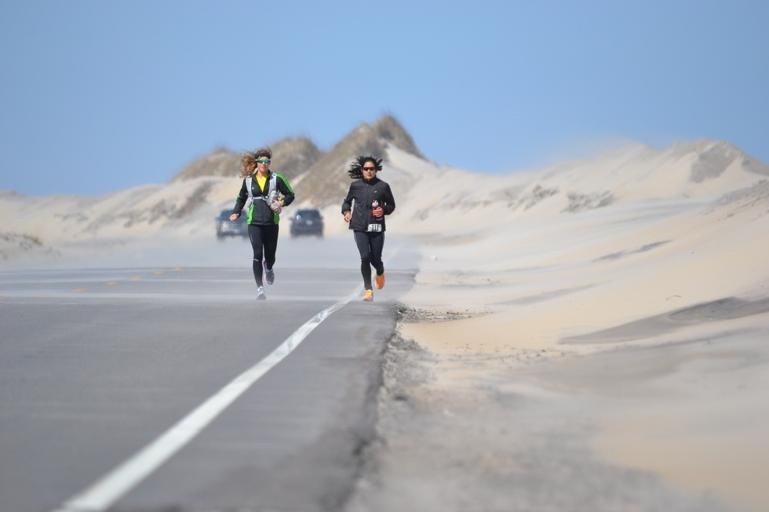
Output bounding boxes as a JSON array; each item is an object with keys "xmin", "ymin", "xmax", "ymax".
[
  {"xmin": 229, "ymin": 144, "xmax": 295, "ymax": 301},
  {"xmin": 341, "ymin": 153, "xmax": 396, "ymax": 302}
]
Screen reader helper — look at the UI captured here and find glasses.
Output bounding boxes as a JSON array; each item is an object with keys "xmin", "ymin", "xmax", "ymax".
[
  {"xmin": 364, "ymin": 167, "xmax": 375, "ymax": 171},
  {"xmin": 256, "ymin": 159, "xmax": 270, "ymax": 163}
]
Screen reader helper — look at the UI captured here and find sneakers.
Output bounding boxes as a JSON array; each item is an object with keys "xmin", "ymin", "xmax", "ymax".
[
  {"xmin": 257, "ymin": 286, "xmax": 266, "ymax": 300},
  {"xmin": 264, "ymin": 260, "xmax": 274, "ymax": 285},
  {"xmin": 362, "ymin": 289, "xmax": 373, "ymax": 301},
  {"xmin": 376, "ymin": 274, "xmax": 384, "ymax": 288}
]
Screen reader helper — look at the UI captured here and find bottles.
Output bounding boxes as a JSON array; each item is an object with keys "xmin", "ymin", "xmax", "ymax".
[{"xmin": 372, "ymin": 201, "xmax": 379, "ymax": 213}]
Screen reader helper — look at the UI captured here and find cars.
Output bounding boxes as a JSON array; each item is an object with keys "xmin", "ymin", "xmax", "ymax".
[
  {"xmin": 289, "ymin": 209, "xmax": 323, "ymax": 235},
  {"xmin": 216, "ymin": 210, "xmax": 248, "ymax": 237}
]
